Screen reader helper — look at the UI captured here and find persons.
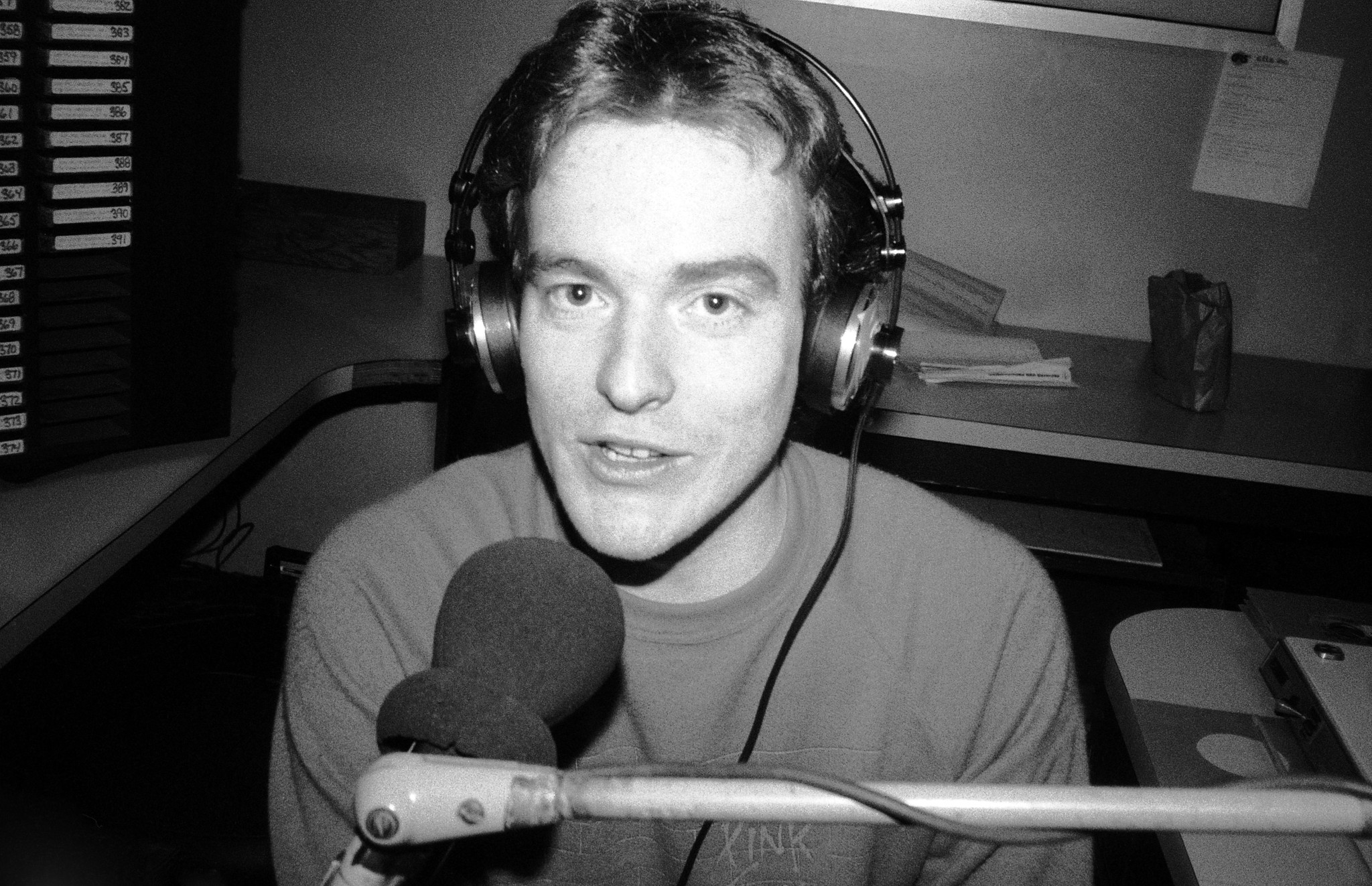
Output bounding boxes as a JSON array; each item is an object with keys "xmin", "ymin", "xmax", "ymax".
[{"xmin": 263, "ymin": 4, "xmax": 1098, "ymax": 883}]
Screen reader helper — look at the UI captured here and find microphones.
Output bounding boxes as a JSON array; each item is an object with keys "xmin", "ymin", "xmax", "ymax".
[{"xmin": 309, "ymin": 528, "xmax": 628, "ymax": 886}]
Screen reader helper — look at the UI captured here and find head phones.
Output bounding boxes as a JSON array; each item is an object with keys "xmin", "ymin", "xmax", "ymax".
[{"xmin": 436, "ymin": 6, "xmax": 912, "ymax": 418}]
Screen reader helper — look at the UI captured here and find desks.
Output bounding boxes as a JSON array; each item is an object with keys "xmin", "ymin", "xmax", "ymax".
[
  {"xmin": 853, "ymin": 321, "xmax": 1372, "ymax": 501},
  {"xmin": 0, "ymin": 249, "xmax": 457, "ymax": 668}
]
